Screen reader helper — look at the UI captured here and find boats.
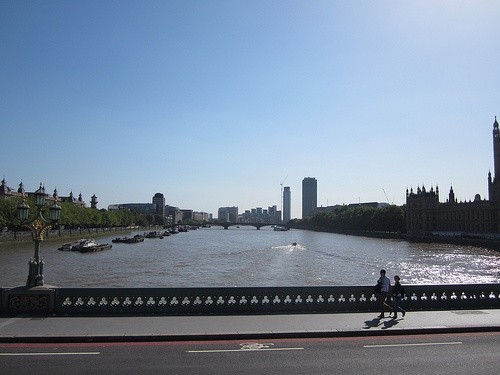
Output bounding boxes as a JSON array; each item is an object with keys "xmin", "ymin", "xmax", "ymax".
[{"xmin": 57, "ymin": 225, "xmax": 197, "ymax": 253}]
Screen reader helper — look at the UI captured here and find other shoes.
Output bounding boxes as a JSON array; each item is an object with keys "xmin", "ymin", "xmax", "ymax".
[
  {"xmin": 390, "ymin": 309, "xmax": 393, "ymax": 315},
  {"xmin": 392, "ymin": 316, "xmax": 397, "ymax": 318},
  {"xmin": 378, "ymin": 316, "xmax": 383, "ymax": 317},
  {"xmin": 402, "ymin": 312, "xmax": 405, "ymax": 317}
]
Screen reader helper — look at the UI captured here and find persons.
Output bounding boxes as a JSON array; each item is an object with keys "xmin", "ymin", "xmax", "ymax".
[
  {"xmin": 391, "ymin": 275, "xmax": 406, "ymax": 318},
  {"xmin": 377, "ymin": 269, "xmax": 394, "ymax": 317}
]
